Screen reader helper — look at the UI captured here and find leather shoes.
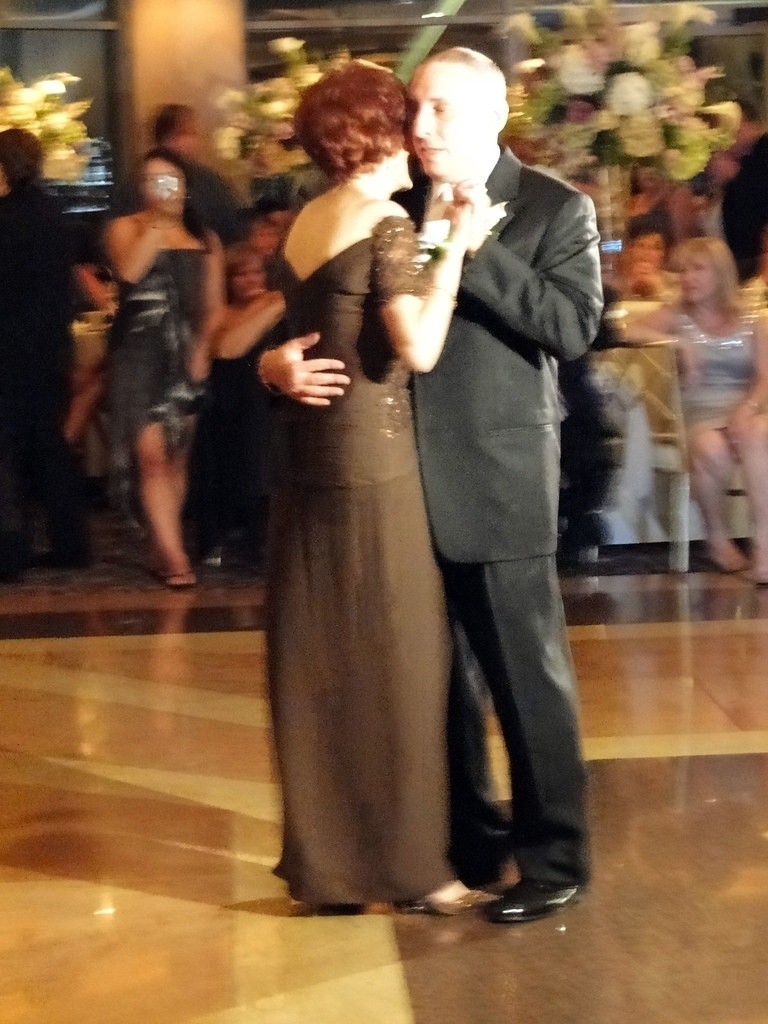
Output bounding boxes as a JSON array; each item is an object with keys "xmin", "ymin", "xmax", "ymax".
[{"xmin": 482, "ymin": 879, "xmax": 575, "ymax": 921}]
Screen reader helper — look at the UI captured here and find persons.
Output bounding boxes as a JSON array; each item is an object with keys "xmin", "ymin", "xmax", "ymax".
[
  {"xmin": 0, "ymin": 99, "xmax": 768, "ymax": 584},
  {"xmin": 238, "ymin": 45, "xmax": 608, "ymax": 920},
  {"xmin": 252, "ymin": 59, "xmax": 506, "ymax": 916}
]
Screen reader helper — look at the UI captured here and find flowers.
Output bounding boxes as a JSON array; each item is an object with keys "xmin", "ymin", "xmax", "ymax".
[
  {"xmin": 493, "ymin": 2, "xmax": 740, "ymax": 187},
  {"xmin": 0, "ymin": 64, "xmax": 99, "ymax": 178},
  {"xmin": 214, "ymin": 31, "xmax": 353, "ymax": 200}
]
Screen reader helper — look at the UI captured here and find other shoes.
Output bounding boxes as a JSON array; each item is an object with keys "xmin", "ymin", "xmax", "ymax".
[{"xmin": 423, "ymin": 876, "xmax": 504, "ymax": 913}]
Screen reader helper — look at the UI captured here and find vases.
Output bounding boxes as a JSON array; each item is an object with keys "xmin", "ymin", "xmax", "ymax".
[{"xmin": 580, "ymin": 160, "xmax": 635, "ymax": 276}]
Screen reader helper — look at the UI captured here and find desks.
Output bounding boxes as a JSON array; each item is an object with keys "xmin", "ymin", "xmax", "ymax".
[{"xmin": 71, "ymin": 310, "xmax": 125, "ymax": 476}]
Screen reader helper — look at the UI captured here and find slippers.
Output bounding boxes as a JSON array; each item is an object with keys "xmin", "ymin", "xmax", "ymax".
[{"xmin": 154, "ymin": 568, "xmax": 201, "ymax": 588}]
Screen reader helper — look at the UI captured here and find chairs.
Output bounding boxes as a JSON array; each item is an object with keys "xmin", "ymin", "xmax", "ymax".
[
  {"xmin": 576, "ymin": 360, "xmax": 652, "ymax": 559},
  {"xmin": 607, "ymin": 297, "xmax": 756, "ymax": 543}
]
